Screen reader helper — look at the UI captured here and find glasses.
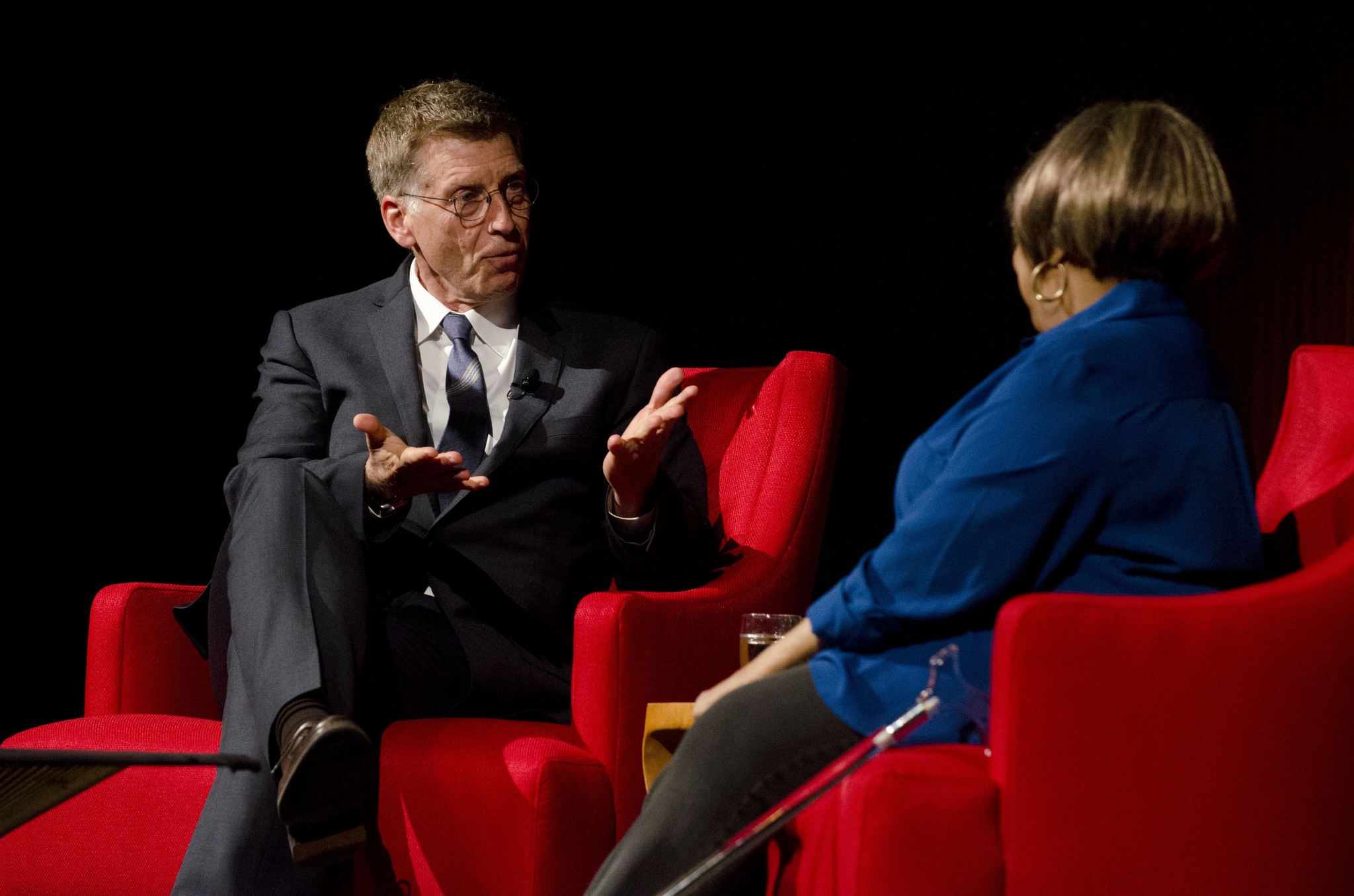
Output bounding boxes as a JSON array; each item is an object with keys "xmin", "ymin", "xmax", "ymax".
[{"xmin": 396, "ymin": 176, "xmax": 539, "ymax": 220}]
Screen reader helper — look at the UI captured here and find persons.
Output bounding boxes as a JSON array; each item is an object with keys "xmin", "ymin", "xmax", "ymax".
[
  {"xmin": 584, "ymin": 99, "xmax": 1264, "ymax": 896},
  {"xmin": 167, "ymin": 80, "xmax": 708, "ymax": 894}
]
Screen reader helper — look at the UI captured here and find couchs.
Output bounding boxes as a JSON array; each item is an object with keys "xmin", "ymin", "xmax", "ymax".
[
  {"xmin": 761, "ymin": 348, "xmax": 1354, "ymax": 896},
  {"xmin": 80, "ymin": 344, "xmax": 836, "ymax": 896}
]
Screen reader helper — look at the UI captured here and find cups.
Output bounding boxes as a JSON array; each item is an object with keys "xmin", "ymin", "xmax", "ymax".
[{"xmin": 741, "ymin": 612, "xmax": 804, "ymax": 669}]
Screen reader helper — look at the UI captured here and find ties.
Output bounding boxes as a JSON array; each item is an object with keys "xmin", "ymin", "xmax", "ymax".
[{"xmin": 430, "ymin": 313, "xmax": 488, "ymax": 519}]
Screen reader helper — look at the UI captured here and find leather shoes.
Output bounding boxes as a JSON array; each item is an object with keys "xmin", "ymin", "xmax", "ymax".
[{"xmin": 277, "ymin": 715, "xmax": 372, "ymax": 871}]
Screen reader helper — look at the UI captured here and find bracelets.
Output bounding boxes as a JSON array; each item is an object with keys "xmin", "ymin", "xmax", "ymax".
[{"xmin": 368, "ymin": 496, "xmax": 411, "ymax": 520}]
